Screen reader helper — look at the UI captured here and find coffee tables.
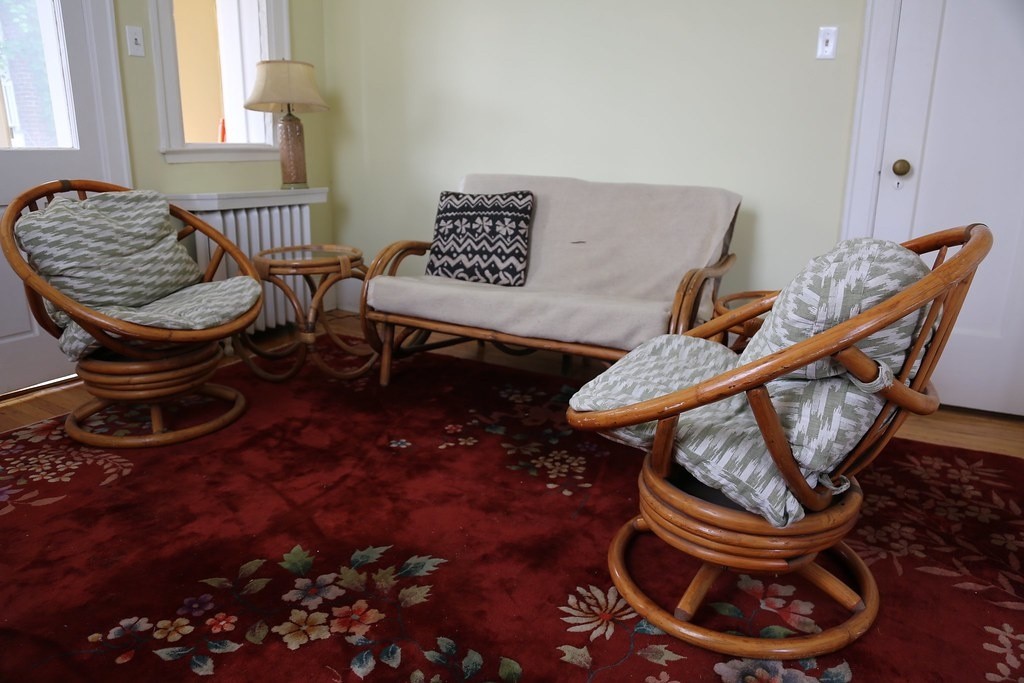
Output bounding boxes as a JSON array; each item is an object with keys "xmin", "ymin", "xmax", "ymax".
[{"xmin": 238, "ymin": 244, "xmax": 386, "ymax": 380}]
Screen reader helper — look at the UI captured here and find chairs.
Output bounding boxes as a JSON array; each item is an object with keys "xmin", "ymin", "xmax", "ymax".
[
  {"xmin": 568, "ymin": 214, "xmax": 994, "ymax": 656},
  {"xmin": 358, "ymin": 169, "xmax": 742, "ymax": 437},
  {"xmin": 2, "ymin": 179, "xmax": 267, "ymax": 448}
]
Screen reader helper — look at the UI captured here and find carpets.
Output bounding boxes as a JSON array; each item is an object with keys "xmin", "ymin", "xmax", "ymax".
[{"xmin": 0, "ymin": 330, "xmax": 1023, "ymax": 682}]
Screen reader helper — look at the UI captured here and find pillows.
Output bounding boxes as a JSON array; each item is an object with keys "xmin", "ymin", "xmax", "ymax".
[{"xmin": 423, "ymin": 188, "xmax": 534, "ymax": 290}]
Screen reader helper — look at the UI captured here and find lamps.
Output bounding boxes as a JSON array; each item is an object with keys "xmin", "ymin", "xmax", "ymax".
[{"xmin": 243, "ymin": 59, "xmax": 333, "ymax": 191}]
